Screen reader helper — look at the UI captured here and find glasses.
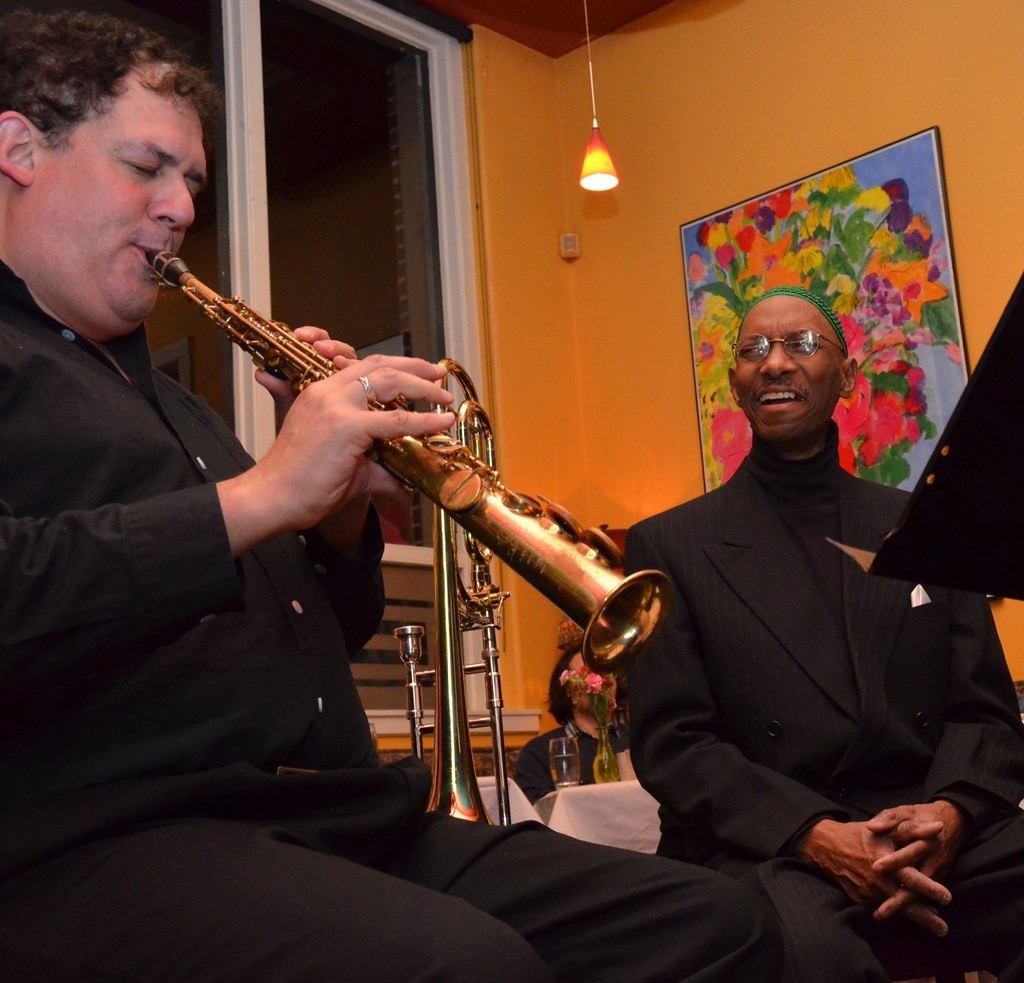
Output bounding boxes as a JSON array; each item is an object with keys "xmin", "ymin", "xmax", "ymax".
[{"xmin": 734, "ymin": 328, "xmax": 843, "ymax": 365}]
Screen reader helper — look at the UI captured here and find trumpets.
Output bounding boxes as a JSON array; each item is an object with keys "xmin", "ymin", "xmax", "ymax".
[{"xmin": 149, "ymin": 250, "xmax": 674, "ymax": 674}]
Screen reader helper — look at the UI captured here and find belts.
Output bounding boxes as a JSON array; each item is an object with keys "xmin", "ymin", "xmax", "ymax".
[{"xmin": 259, "ymin": 764, "xmax": 341, "ymax": 777}]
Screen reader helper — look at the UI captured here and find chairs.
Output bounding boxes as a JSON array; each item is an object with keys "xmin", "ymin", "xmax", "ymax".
[{"xmin": 452, "ymin": 776, "xmax": 545, "ymax": 825}]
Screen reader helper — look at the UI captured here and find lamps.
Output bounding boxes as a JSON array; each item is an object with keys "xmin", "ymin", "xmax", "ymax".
[{"xmin": 579, "ymin": 0, "xmax": 619, "ymax": 191}]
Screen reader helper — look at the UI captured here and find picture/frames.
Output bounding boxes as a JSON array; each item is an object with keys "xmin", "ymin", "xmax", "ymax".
[{"xmin": 679, "ymin": 125, "xmax": 1004, "ymax": 601}]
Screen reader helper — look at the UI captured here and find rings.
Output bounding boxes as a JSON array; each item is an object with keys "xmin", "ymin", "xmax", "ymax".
[{"xmin": 358, "ymin": 376, "xmax": 377, "ymax": 403}]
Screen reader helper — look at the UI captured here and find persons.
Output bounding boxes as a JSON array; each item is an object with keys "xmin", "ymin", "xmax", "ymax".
[
  {"xmin": 517, "ymin": 644, "xmax": 629, "ymax": 808},
  {"xmin": 622, "ymin": 285, "xmax": 1024, "ymax": 983},
  {"xmin": 0, "ymin": 3, "xmax": 786, "ymax": 983}
]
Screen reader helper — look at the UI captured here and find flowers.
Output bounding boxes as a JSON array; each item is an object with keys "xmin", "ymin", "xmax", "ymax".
[{"xmin": 559, "ymin": 666, "xmax": 617, "ymax": 726}]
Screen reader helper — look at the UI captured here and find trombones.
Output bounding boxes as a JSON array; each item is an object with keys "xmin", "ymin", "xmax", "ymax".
[{"xmin": 393, "ymin": 356, "xmax": 511, "ymax": 829}]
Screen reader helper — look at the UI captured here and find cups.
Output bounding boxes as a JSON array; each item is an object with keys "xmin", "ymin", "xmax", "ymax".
[{"xmin": 549, "ymin": 737, "xmax": 581, "ymax": 790}]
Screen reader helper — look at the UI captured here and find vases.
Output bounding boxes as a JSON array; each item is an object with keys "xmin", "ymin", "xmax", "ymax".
[{"xmin": 594, "ymin": 727, "xmax": 619, "ymax": 784}]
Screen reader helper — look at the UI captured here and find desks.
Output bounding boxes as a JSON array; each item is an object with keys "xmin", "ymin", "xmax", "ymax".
[{"xmin": 534, "ymin": 779, "xmax": 662, "ymax": 854}]
